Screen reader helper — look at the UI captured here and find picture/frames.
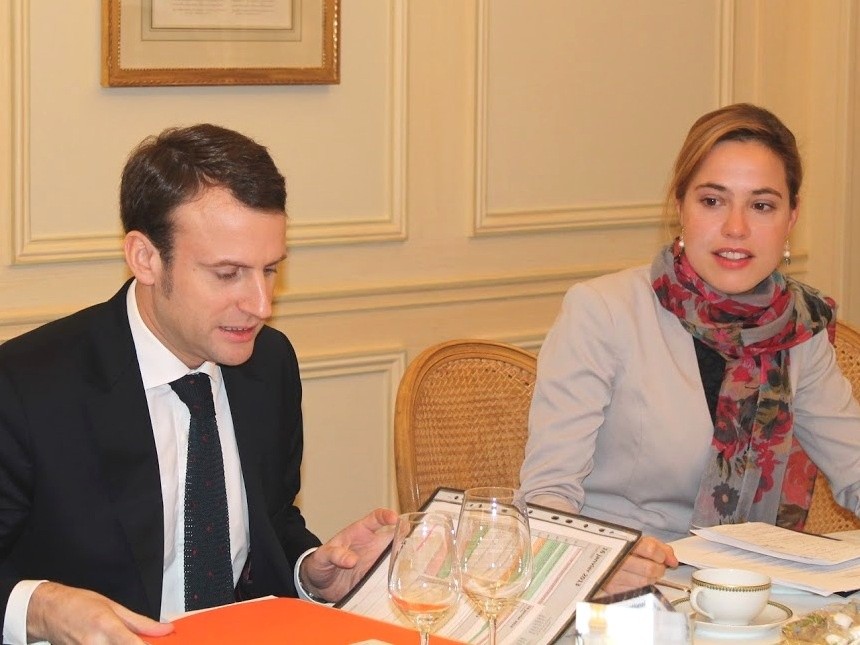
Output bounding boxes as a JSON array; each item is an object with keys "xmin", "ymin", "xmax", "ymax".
[{"xmin": 101, "ymin": 0, "xmax": 340, "ymax": 88}]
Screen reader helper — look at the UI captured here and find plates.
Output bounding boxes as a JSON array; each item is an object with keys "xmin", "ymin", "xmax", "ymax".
[{"xmin": 668, "ymin": 595, "xmax": 794, "ymax": 640}]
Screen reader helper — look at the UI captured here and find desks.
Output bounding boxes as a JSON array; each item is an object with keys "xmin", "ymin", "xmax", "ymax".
[{"xmin": 555, "ymin": 529, "xmax": 860, "ymax": 645}]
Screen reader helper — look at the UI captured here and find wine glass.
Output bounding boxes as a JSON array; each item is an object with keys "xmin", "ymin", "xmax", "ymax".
[
  {"xmin": 387, "ymin": 512, "xmax": 461, "ymax": 645},
  {"xmin": 453, "ymin": 486, "xmax": 533, "ymax": 645}
]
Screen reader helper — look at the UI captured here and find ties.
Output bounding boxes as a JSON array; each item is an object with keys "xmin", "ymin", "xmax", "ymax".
[{"xmin": 168, "ymin": 372, "xmax": 235, "ymax": 613}]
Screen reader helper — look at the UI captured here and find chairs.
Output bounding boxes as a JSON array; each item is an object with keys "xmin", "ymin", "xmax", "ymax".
[
  {"xmin": 394, "ymin": 338, "xmax": 538, "ymax": 511},
  {"xmin": 802, "ymin": 321, "xmax": 860, "ymax": 534}
]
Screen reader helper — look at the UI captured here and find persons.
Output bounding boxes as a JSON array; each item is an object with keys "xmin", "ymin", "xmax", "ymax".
[
  {"xmin": 521, "ymin": 103, "xmax": 860, "ymax": 595},
  {"xmin": 0, "ymin": 123, "xmax": 398, "ymax": 645}
]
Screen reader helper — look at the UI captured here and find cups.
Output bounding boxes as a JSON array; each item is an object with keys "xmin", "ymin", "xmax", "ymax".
[{"xmin": 689, "ymin": 568, "xmax": 772, "ymax": 625}]
